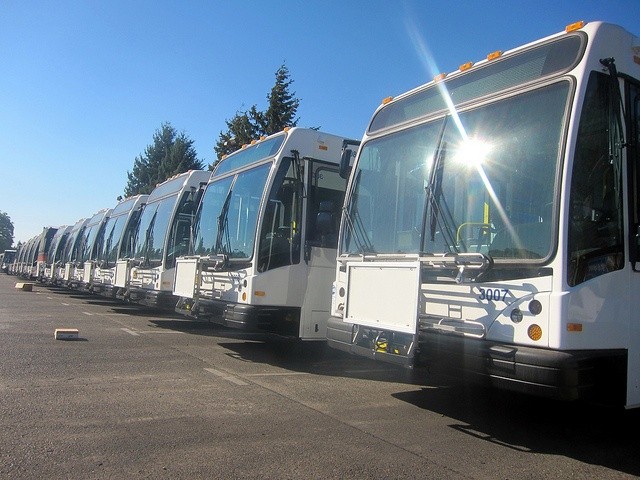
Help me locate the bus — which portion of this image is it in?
[1,227,58,280]
[173,127,359,341]
[325,20,640,421]
[60,218,91,293]
[43,225,74,286]
[92,194,150,300]
[124,169,212,310]
[71,209,114,298]
